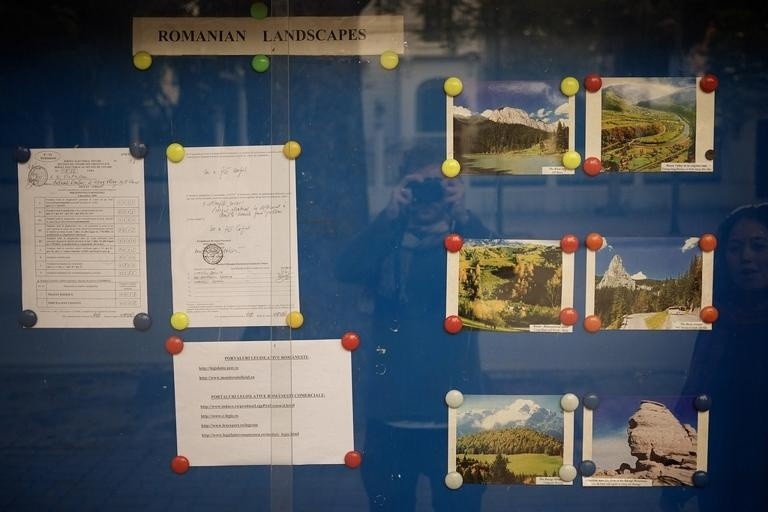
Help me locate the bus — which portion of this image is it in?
[667,306,686,315]
